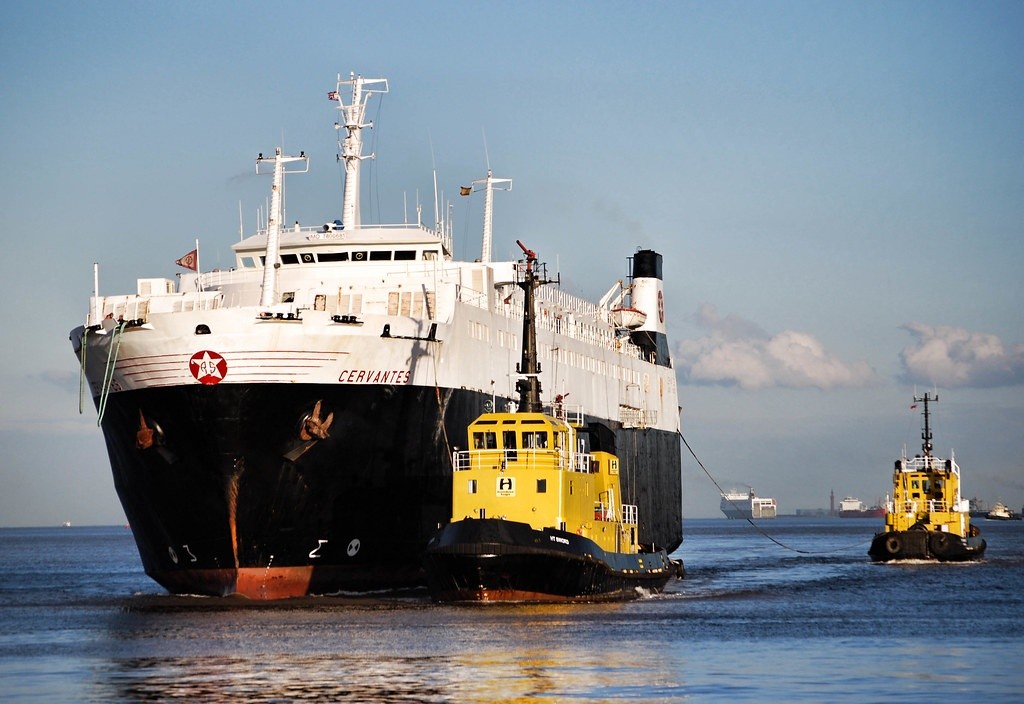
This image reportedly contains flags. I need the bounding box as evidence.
[328,91,339,101]
[459,187,472,196]
[176,249,199,271]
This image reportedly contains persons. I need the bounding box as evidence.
[106,313,125,330]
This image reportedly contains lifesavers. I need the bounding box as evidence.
[932,533,951,556]
[675,559,686,579]
[886,536,901,553]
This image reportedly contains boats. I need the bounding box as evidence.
[985,502,1021,520]
[68,70,685,599]
[419,240,685,605]
[720,486,778,518]
[867,380,987,563]
[838,492,893,518]
